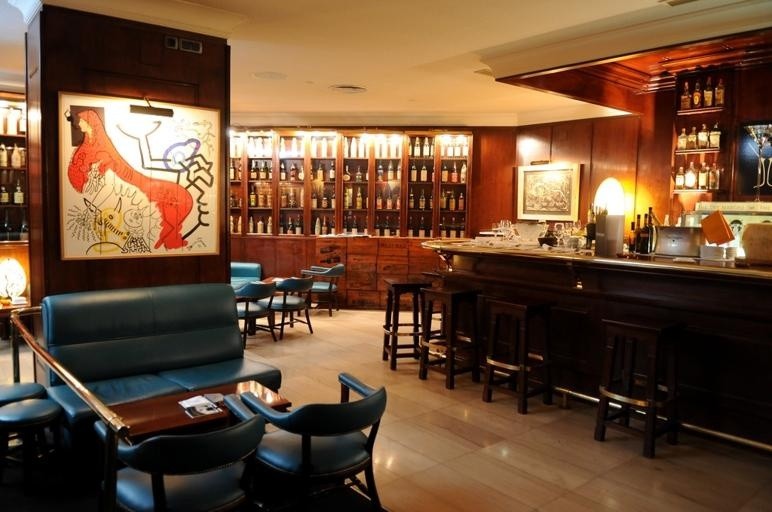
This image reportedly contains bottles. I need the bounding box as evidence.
[463,138,468,158]
[7,106,17,134]
[440,143,444,158]
[684,162,697,189]
[703,76,712,107]
[258,192,265,207]
[366,194,369,211]
[408,194,414,209]
[420,161,427,182]
[287,216,294,234]
[449,188,456,211]
[230,216,233,233]
[356,187,363,209]
[251,160,257,180]
[230,192,235,207]
[230,139,235,155]
[343,216,348,235]
[260,161,266,180]
[289,162,296,181]
[414,137,420,158]
[351,138,356,157]
[455,143,461,158]
[281,191,286,207]
[386,193,393,210]
[395,216,401,236]
[714,78,725,106]
[247,137,256,156]
[364,216,368,235]
[331,185,335,209]
[20,208,29,241]
[351,215,358,235]
[687,127,696,149]
[635,214,642,258]
[281,163,285,181]
[267,192,272,207]
[6,147,12,167]
[395,195,400,209]
[322,216,327,235]
[11,143,21,169]
[365,169,368,182]
[430,195,433,210]
[449,217,457,238]
[344,193,348,209]
[264,138,272,156]
[431,141,434,158]
[248,216,254,233]
[458,192,464,211]
[710,121,720,148]
[238,160,241,180]
[374,216,380,236]
[640,214,651,262]
[432,167,435,182]
[296,214,301,234]
[358,140,364,158]
[300,188,304,208]
[311,138,317,157]
[256,138,264,156]
[441,161,448,183]
[376,192,384,209]
[343,138,348,158]
[381,141,387,157]
[461,162,467,183]
[384,216,390,236]
[408,137,412,158]
[270,161,272,179]
[408,216,413,237]
[19,148,26,166]
[629,222,637,258]
[330,160,336,181]
[292,138,297,157]
[321,198,327,208]
[709,162,720,190]
[451,161,458,182]
[387,160,394,182]
[418,215,425,238]
[678,128,687,148]
[440,189,446,210]
[347,211,351,231]
[585,210,596,257]
[356,166,362,181]
[238,216,241,233]
[397,160,401,181]
[675,166,685,189]
[440,216,446,237]
[1,143,7,166]
[18,109,26,135]
[14,178,24,204]
[298,165,304,180]
[322,138,327,157]
[699,162,708,190]
[460,218,465,238]
[239,197,242,207]
[680,81,691,110]
[249,192,256,207]
[343,165,351,181]
[237,139,241,156]
[693,80,701,108]
[311,192,317,208]
[374,140,380,158]
[288,188,296,207]
[279,214,283,234]
[390,143,396,157]
[377,161,383,182]
[346,186,353,208]
[423,137,430,158]
[3,207,13,241]
[317,163,323,181]
[310,165,313,181]
[0,187,9,204]
[280,138,285,156]
[648,207,658,255]
[418,188,426,209]
[332,138,335,157]
[399,141,402,158]
[698,123,710,149]
[230,158,234,180]
[257,216,264,233]
[366,141,368,157]
[330,216,334,235]
[300,140,305,157]
[267,216,272,232]
[430,227,433,237]
[314,217,320,235]
[411,161,417,182]
[447,143,453,158]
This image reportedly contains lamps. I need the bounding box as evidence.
[746,125,772,202]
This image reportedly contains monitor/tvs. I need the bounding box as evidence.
[655,226,704,259]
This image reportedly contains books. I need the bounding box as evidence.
[184,401,223,419]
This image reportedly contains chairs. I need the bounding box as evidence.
[95,394,266,510]
[301,264,344,316]
[233,281,276,348]
[257,275,314,340]
[243,372,387,511]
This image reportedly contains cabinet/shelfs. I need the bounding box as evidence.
[671,71,733,198]
[231,128,472,307]
[0,92,32,243]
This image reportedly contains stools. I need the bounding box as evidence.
[594,311,679,457]
[419,283,481,389]
[383,277,431,370]
[482,294,554,415]
[0,381,45,400]
[1,400,67,468]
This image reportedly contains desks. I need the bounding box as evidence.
[106,381,292,444]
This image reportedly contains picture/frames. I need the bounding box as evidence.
[517,165,582,223]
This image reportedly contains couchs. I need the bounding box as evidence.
[40,283,285,418]
[231,263,261,289]
